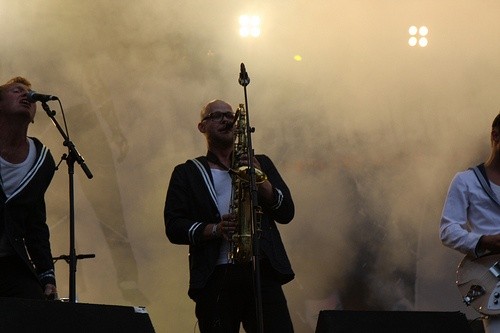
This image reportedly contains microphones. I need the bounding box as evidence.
[239,63,250,86]
[26,92,58,103]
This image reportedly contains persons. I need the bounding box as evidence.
[0,76,57,299]
[164,99,296,333]
[440,113,500,333]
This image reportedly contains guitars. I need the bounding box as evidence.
[457,248,500,314]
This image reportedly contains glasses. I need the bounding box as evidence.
[201,111,236,122]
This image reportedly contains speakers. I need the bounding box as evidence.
[0,295,155,333]
[315,310,474,333]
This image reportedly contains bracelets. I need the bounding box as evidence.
[212,223,217,236]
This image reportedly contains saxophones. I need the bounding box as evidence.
[223,103,267,276]
